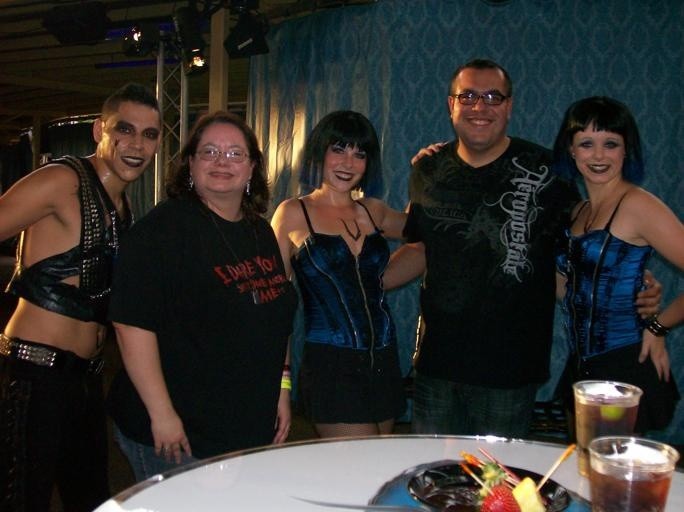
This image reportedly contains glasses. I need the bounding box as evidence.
[452,91,506,105]
[199,144,247,163]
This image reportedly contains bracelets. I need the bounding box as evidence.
[279,364,291,391]
[646,317,671,338]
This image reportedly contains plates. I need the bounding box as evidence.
[408,464,572,512]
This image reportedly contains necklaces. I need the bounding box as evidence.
[208,200,262,307]
[584,205,602,234]
[337,216,363,243]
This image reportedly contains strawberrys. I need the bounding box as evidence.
[481,486,520,511]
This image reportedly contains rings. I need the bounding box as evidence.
[163,447,172,454]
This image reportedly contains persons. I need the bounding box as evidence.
[0,83,160,510]
[112,104,296,484]
[271,110,448,439]
[383,57,666,441]
[554,95,684,453]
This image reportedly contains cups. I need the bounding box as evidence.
[587,434,680,512]
[572,380,644,479]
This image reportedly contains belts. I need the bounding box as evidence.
[1,334,105,376]
